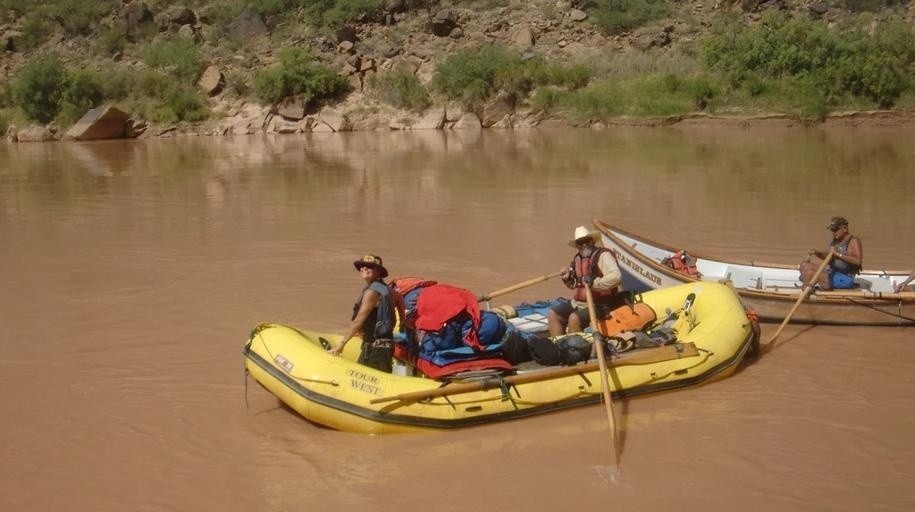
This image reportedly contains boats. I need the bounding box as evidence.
[242,280,755,436]
[593,218,910,325]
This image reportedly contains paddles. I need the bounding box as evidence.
[581,276,620,469]
[368,341,700,404]
[763,252,834,348]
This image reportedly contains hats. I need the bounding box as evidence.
[826,217,848,230]
[354,254,388,277]
[566,226,601,247]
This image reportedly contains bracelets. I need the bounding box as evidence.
[839,253,843,258]
[340,339,345,345]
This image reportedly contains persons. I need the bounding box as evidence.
[547,226,623,339]
[800,216,864,288]
[329,251,406,373]
